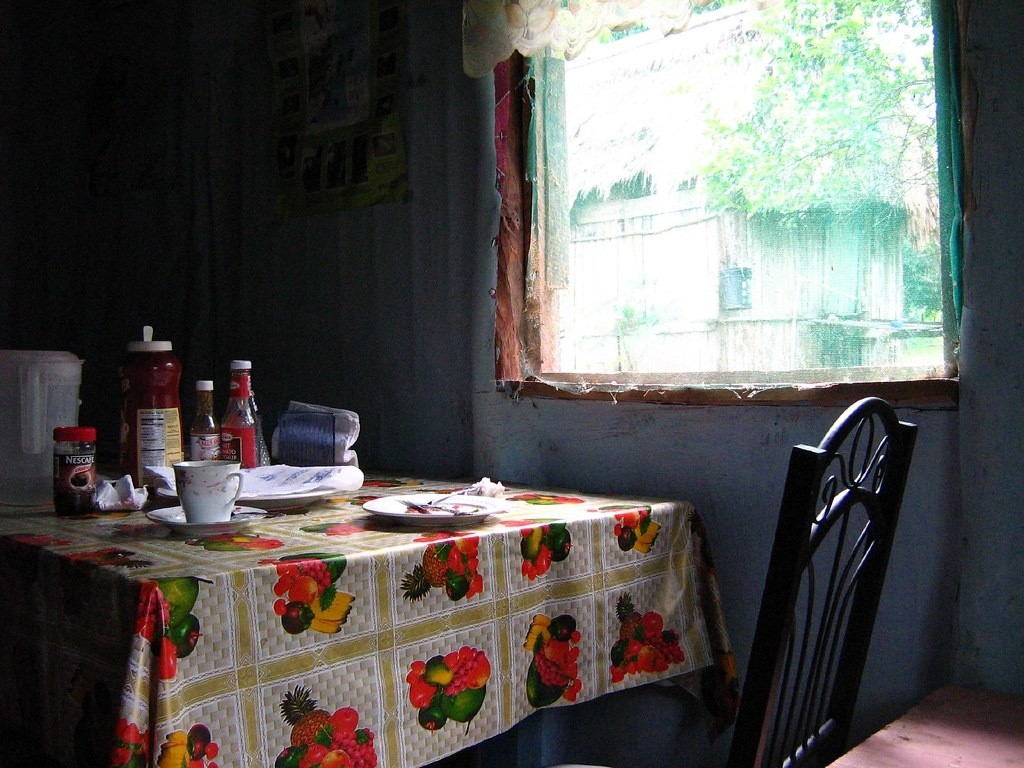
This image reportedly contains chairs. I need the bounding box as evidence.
[721,395,919,768]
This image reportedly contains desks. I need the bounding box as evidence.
[0,465,739,768]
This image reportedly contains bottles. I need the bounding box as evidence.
[220,360,270,468]
[189,381,220,461]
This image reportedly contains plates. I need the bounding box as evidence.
[156,465,364,512]
[145,505,268,536]
[362,494,510,526]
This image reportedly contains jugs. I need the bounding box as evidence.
[0,349,86,506]
